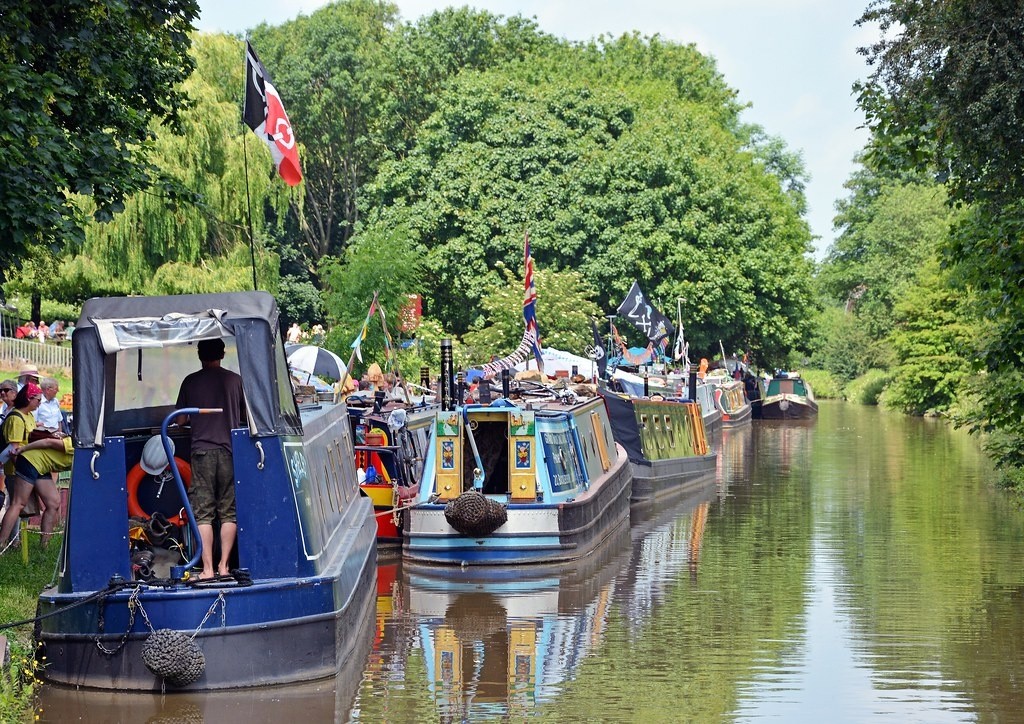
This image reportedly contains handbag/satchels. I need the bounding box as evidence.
[28,429,60,443]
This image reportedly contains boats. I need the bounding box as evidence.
[348,296,821,568]
[34,578,381,724]
[400,514,635,723]
[32,290,380,693]
[631,476,719,544]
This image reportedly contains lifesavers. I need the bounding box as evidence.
[126,457,192,528]
[648,378,665,385]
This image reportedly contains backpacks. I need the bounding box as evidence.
[0,411,26,454]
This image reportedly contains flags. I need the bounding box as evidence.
[592,321,607,379]
[523,233,543,364]
[241,39,302,187]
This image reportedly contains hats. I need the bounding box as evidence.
[13,365,44,379]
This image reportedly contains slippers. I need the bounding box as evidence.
[219,573,232,579]
[187,576,214,584]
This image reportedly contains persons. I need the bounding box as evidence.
[731,367,766,401]
[0,365,75,551]
[175,338,247,582]
[359,372,407,402]
[24,320,76,346]
[286,322,324,343]
[469,377,479,392]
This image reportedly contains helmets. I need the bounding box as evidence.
[140,434,175,475]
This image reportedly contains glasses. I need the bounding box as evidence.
[33,397,41,402]
[31,377,38,381]
[0,388,12,393]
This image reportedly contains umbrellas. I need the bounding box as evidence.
[285,345,347,385]
